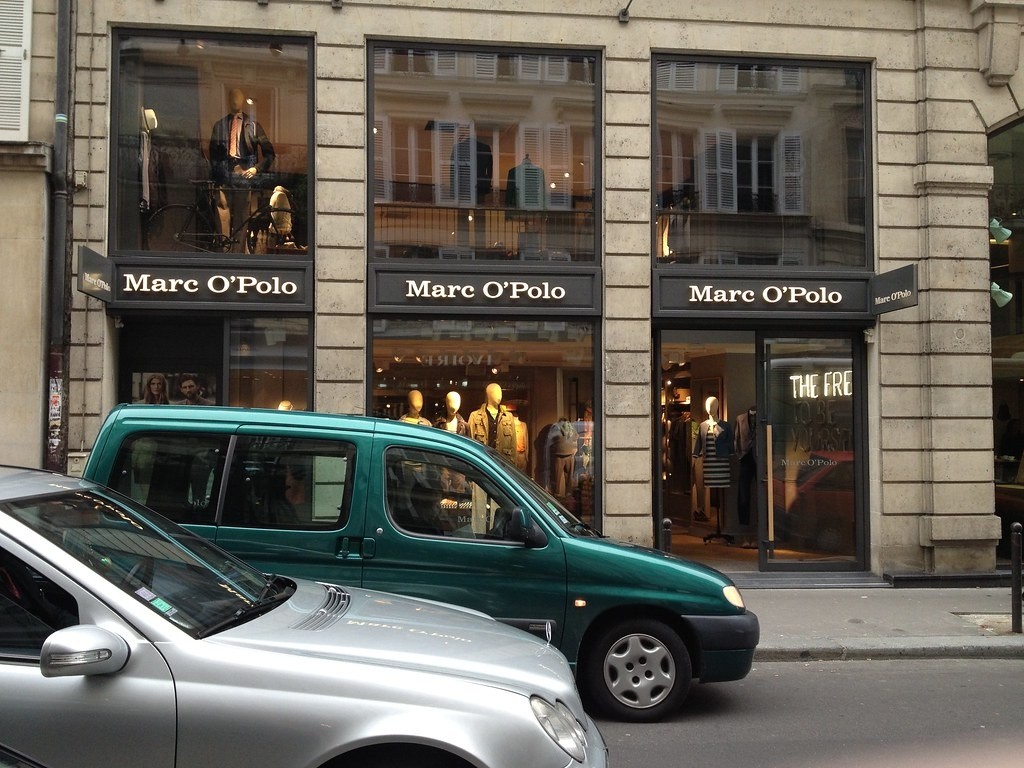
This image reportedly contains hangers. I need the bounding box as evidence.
[679,412,692,421]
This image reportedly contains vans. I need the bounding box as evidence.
[64,402,760,724]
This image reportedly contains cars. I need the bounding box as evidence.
[0,464,611,768]
[751,450,855,552]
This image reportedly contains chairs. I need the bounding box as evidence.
[238,454,301,525]
[410,479,443,530]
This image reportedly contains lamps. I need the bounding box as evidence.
[989,216,1012,243]
[661,352,672,370]
[668,351,686,366]
[989,282,1013,307]
[491,364,503,374]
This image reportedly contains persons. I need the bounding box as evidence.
[210,88,292,254]
[450,126,493,247]
[692,397,758,525]
[277,400,306,505]
[131,373,216,509]
[504,154,544,221]
[548,417,579,499]
[580,445,593,473]
[395,383,529,496]
